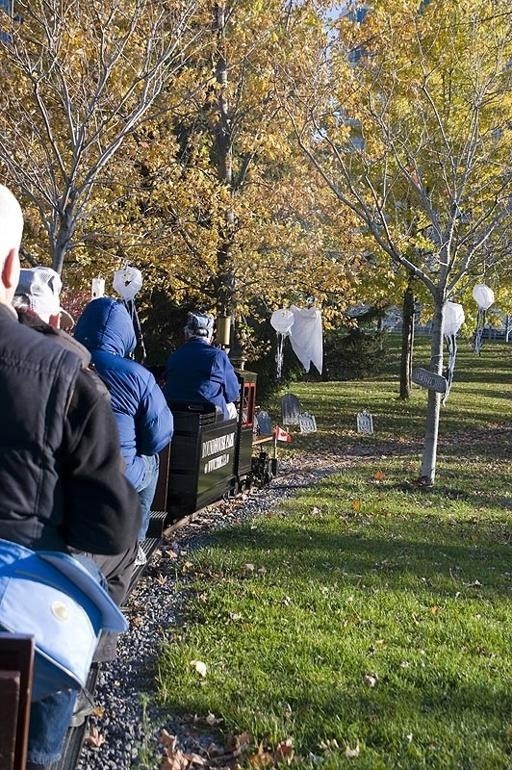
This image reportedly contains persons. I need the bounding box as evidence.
[21,268,138,725]
[1,183,138,769]
[72,298,173,566]
[163,312,240,423]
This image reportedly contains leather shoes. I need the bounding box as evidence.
[70,690,95,727]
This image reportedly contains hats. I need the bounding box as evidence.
[17,265,74,329]
[187,314,214,336]
